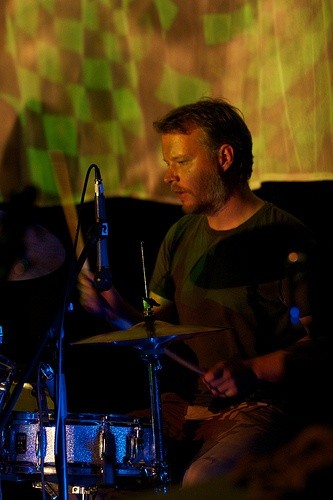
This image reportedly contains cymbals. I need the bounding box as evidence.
[0,220,66,281]
[0,381,56,411]
[70,319,233,345]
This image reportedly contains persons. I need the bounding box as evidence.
[79,101,312,487]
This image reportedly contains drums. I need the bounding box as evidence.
[1,414,166,475]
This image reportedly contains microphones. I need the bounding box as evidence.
[94,168,112,290]
